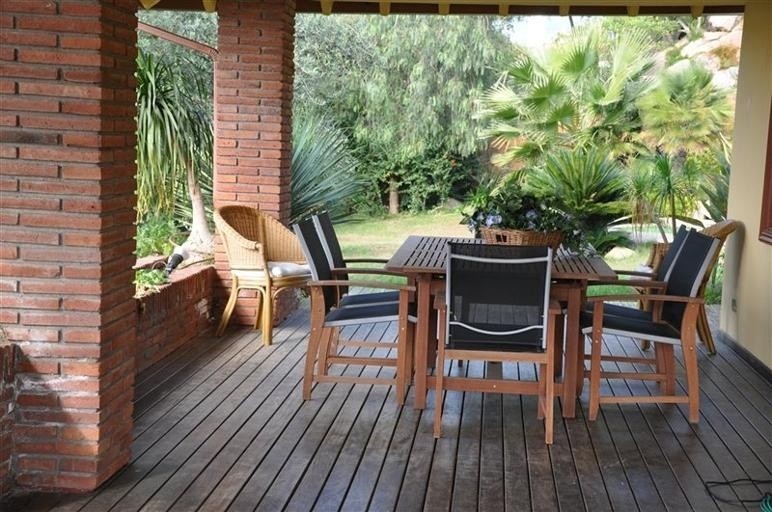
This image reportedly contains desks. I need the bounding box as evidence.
[385,235,619,417]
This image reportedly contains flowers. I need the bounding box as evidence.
[461,171,579,252]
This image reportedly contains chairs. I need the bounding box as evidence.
[293,220,417,405]
[639,219,737,354]
[576,228,719,423]
[587,225,694,390]
[214,205,311,345]
[313,211,417,356]
[433,245,561,444]
